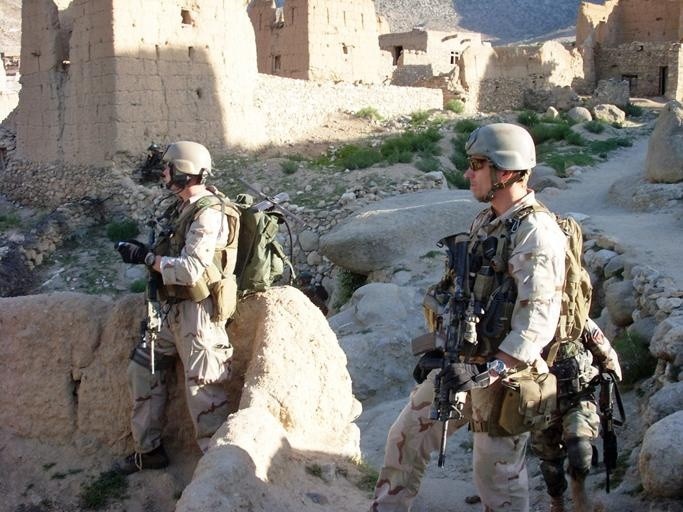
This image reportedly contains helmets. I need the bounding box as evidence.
[463,122,537,172]
[161,139,212,176]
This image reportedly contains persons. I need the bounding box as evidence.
[111,141,238,476]
[528,315,621,511]
[368,123,566,510]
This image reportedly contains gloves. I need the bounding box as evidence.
[435,360,491,394]
[114,236,149,264]
[412,351,445,386]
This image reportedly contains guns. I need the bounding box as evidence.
[411,233,494,467]
[599,371,618,495]
[129,219,177,375]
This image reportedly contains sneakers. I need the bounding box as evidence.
[116,443,169,475]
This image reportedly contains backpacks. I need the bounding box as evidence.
[169,191,296,299]
[499,204,595,348]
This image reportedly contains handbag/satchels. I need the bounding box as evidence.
[208,274,238,321]
[499,361,562,437]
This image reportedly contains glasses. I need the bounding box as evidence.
[464,155,489,171]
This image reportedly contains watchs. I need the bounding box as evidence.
[490,359,508,374]
